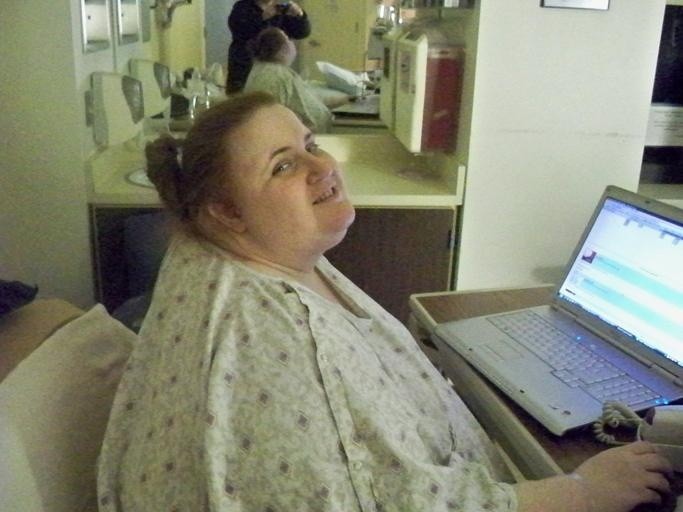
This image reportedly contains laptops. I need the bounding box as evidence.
[432,186,683,437]
[331,96,379,119]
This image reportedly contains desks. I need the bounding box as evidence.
[404,285,683,512]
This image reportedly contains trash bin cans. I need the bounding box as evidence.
[112,290,154,336]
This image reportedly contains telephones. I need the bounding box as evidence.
[636,405,683,471]
[358,94,380,107]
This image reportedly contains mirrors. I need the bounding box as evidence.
[72,0,401,134]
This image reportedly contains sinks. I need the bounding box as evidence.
[126,167,155,189]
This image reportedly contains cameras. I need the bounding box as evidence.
[275,2,290,12]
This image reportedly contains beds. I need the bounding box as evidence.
[0,296,137,511]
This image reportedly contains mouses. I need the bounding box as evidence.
[630,487,677,511]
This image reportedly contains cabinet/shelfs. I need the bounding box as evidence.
[93,209,459,314]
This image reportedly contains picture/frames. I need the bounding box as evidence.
[540,0,610,11]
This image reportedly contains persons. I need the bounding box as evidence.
[224,0,334,133]
[98,93,675,511]
